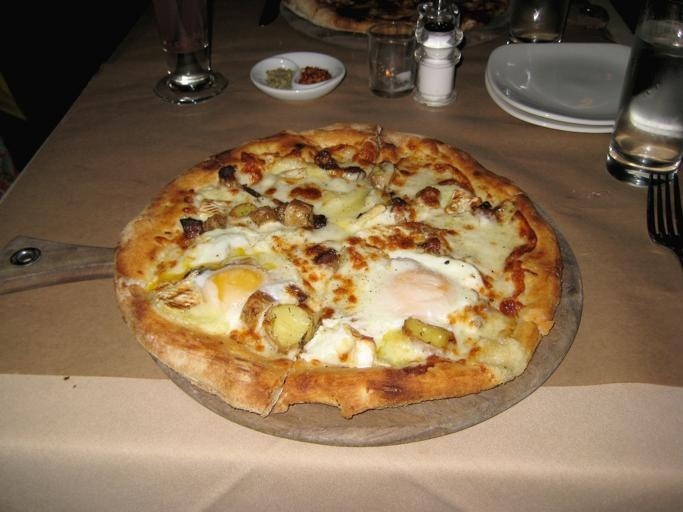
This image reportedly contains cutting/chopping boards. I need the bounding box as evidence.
[1,125,585,448]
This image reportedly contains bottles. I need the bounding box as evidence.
[414,22,465,110]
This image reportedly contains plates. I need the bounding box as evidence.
[483,42,641,136]
[246,50,346,101]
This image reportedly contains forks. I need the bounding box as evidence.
[645,168,682,281]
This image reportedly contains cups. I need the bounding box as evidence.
[601,2,682,186]
[152,0,229,104]
[366,21,418,98]
[502,0,570,44]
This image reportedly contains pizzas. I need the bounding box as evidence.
[281,0,510,38]
[114,121,563,418]
